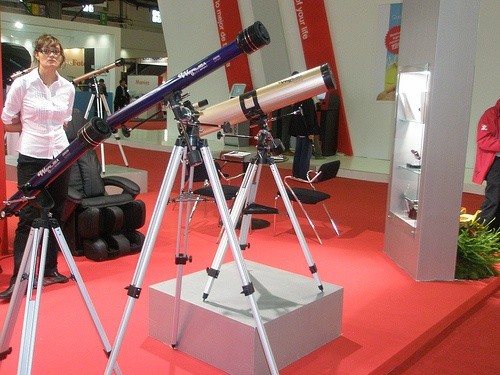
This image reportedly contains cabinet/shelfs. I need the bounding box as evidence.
[384,0,481,282]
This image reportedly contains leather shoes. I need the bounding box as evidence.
[44,271,69,283]
[0,282,27,298]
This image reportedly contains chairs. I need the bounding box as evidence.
[273,160,342,245]
[63,108,147,262]
[187,160,241,233]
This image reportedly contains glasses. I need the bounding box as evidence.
[38,47,63,54]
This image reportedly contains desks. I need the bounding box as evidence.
[221,151,289,231]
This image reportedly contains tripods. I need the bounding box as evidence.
[202,114,324,301]
[0,186,123,375]
[102,89,279,375]
[84,76,129,175]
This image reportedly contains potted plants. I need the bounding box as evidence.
[454,206,500,280]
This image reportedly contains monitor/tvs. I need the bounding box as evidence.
[230,84,246,97]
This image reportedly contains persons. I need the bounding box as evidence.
[113,79,130,126]
[281,71,325,182]
[470,98,500,232]
[0,31,76,298]
[93,79,107,119]
